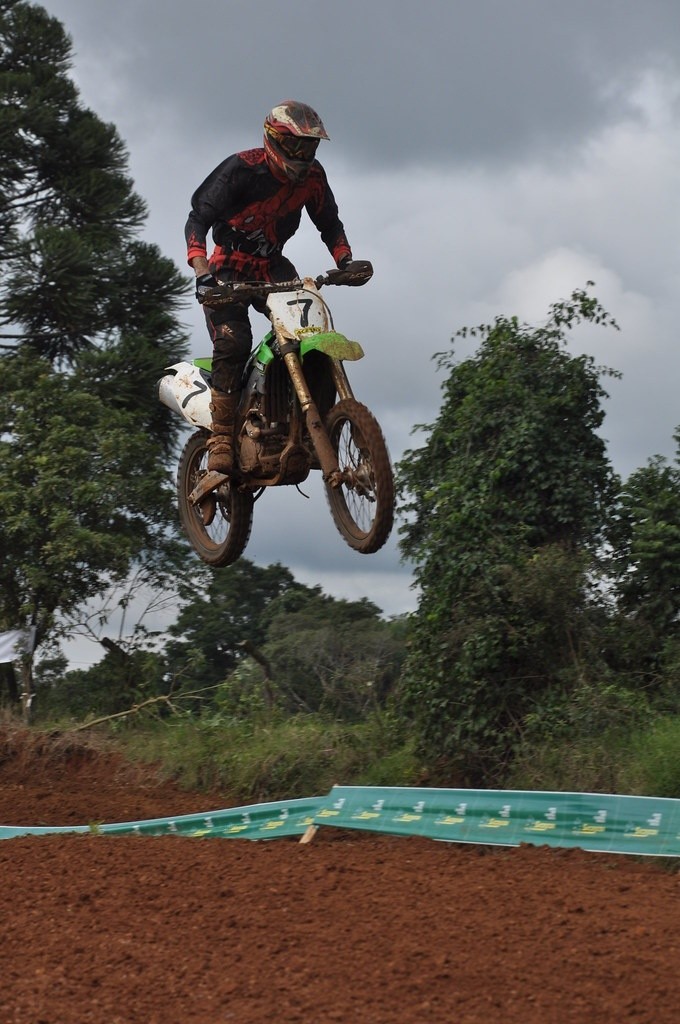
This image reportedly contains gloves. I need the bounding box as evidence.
[340,260,353,272]
[196,274,222,305]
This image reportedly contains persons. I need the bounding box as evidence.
[184,101,373,470]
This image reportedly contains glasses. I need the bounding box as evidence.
[279,135,319,159]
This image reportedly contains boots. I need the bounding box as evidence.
[206,388,238,473]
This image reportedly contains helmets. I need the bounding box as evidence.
[263,101,330,185]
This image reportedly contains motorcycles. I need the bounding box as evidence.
[158,264,399,569]
